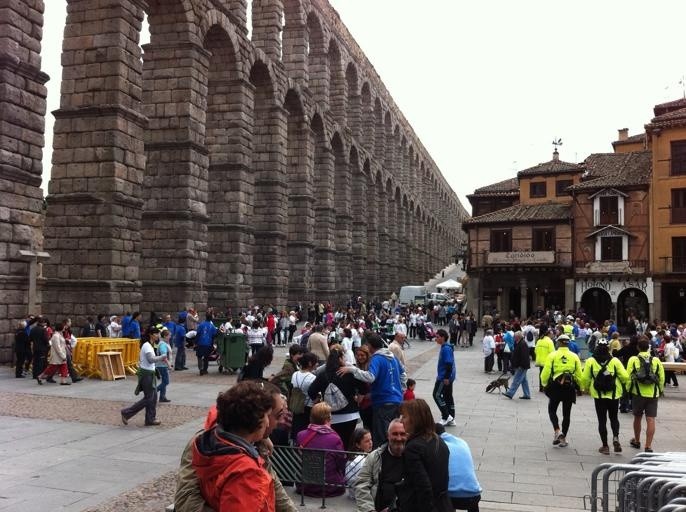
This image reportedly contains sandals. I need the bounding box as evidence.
[37,377,42,385]
[60,382,71,385]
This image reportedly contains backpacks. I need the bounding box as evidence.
[626,338,664,452]
[588,363,625,401]
[546,371,582,405]
[524,327,535,343]
[320,371,348,416]
[288,372,310,413]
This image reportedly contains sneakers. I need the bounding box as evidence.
[613,439,622,453]
[159,398,170,402]
[645,447,653,452]
[438,414,453,426]
[121,410,128,425]
[630,439,641,449]
[599,446,609,454]
[145,419,160,425]
[446,420,456,426]
[553,431,563,445]
[559,438,568,447]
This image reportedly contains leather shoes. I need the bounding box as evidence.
[519,396,531,399]
[182,367,188,369]
[72,377,84,383]
[502,392,512,399]
[174,368,183,371]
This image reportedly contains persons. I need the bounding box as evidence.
[225,317,233,330]
[180,307,188,321]
[272,315,283,346]
[449,291,465,307]
[493,311,534,330]
[502,331,530,400]
[627,311,664,359]
[665,337,678,390]
[584,339,634,457]
[15,321,28,379]
[69,331,78,349]
[31,314,49,379]
[664,321,685,345]
[268,312,275,344]
[470,315,475,348]
[270,340,304,391]
[122,328,167,428]
[299,322,365,348]
[502,324,515,375]
[269,395,290,448]
[290,353,317,409]
[339,329,356,369]
[469,308,473,315]
[121,313,144,341]
[81,317,97,337]
[370,296,394,330]
[388,330,417,399]
[523,320,538,360]
[35,323,72,385]
[463,316,470,348]
[395,316,409,338]
[346,426,372,499]
[290,311,297,341]
[20,315,36,369]
[432,330,458,425]
[482,328,498,374]
[336,329,403,450]
[429,295,451,324]
[294,400,348,497]
[537,334,584,448]
[307,326,330,365]
[458,312,466,343]
[415,306,427,345]
[109,316,120,337]
[97,314,110,337]
[43,318,53,340]
[260,320,268,349]
[396,299,429,318]
[47,318,84,383]
[281,312,290,344]
[311,342,347,374]
[354,418,407,512]
[235,311,255,326]
[175,318,189,371]
[481,311,492,333]
[185,308,198,347]
[495,327,505,370]
[249,322,263,354]
[401,399,450,512]
[166,313,175,362]
[208,305,214,318]
[196,312,218,375]
[226,305,232,317]
[534,301,622,357]
[452,299,460,314]
[248,304,272,324]
[297,294,369,322]
[409,309,418,336]
[434,422,482,512]
[310,347,368,456]
[174,379,295,512]
[354,345,367,373]
[154,315,165,330]
[190,380,276,511]
[448,311,459,350]
[156,330,173,404]
[29,314,42,375]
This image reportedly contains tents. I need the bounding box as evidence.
[436,278,462,293]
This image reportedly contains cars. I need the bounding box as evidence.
[428,293,454,303]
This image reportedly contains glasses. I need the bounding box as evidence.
[269,412,283,420]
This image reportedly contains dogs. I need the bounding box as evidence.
[485,375,511,394]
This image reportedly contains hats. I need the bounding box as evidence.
[555,335,570,341]
[597,338,608,346]
